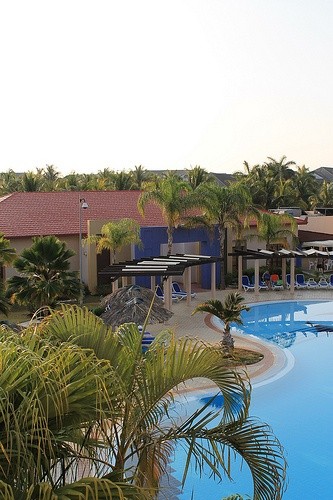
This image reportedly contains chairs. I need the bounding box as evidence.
[286,274,308,289]
[241,271,283,293]
[154,282,196,302]
[307,274,333,288]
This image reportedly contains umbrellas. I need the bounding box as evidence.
[100,285,166,309]
[98,297,174,330]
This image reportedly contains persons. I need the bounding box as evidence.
[263,271,270,291]
[271,273,280,290]
[322,259,325,274]
[324,257,328,270]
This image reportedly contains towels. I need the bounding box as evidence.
[277,279,282,284]
[271,274,278,281]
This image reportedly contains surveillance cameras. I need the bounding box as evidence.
[82,203,88,209]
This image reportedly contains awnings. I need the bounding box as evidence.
[301,240,333,249]
